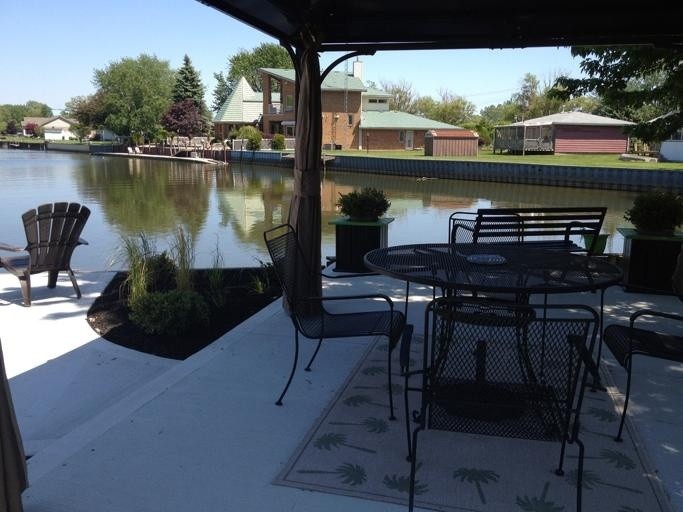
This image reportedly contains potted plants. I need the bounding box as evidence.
[623,187,683,236]
[335,187,392,222]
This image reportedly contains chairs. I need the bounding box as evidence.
[406,296,600,512]
[590,308,683,443]
[262,223,405,421]
[0,202,90,307]
[426,208,609,382]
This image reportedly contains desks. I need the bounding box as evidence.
[362,241,625,417]
